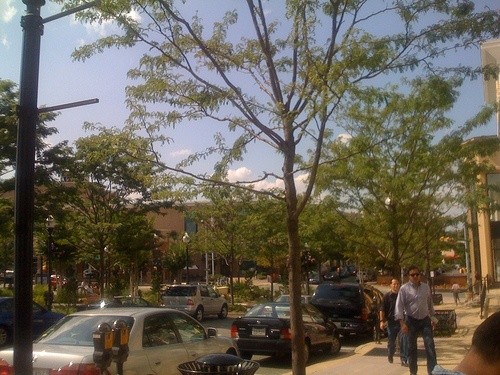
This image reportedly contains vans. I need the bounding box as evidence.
[309,281,388,343]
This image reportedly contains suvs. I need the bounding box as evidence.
[0,270,15,284]
[356,271,368,282]
[50,274,77,291]
[163,282,228,322]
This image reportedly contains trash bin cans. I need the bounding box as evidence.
[433,310,452,336]
[178,353,260,375]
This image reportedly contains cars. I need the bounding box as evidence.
[275,294,311,303]
[0,306,239,375]
[309,265,357,284]
[230,302,342,364]
[86,295,155,308]
[0,296,69,347]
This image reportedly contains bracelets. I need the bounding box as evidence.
[380,319,384,322]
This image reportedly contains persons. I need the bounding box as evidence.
[432,312,500,375]
[394,265,438,375]
[452,281,462,305]
[379,277,409,367]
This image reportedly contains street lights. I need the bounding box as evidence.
[183,232,191,284]
[104,244,114,296]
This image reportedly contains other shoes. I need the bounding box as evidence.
[388,356,393,363]
[401,360,409,367]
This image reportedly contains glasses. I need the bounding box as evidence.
[409,273,419,277]
